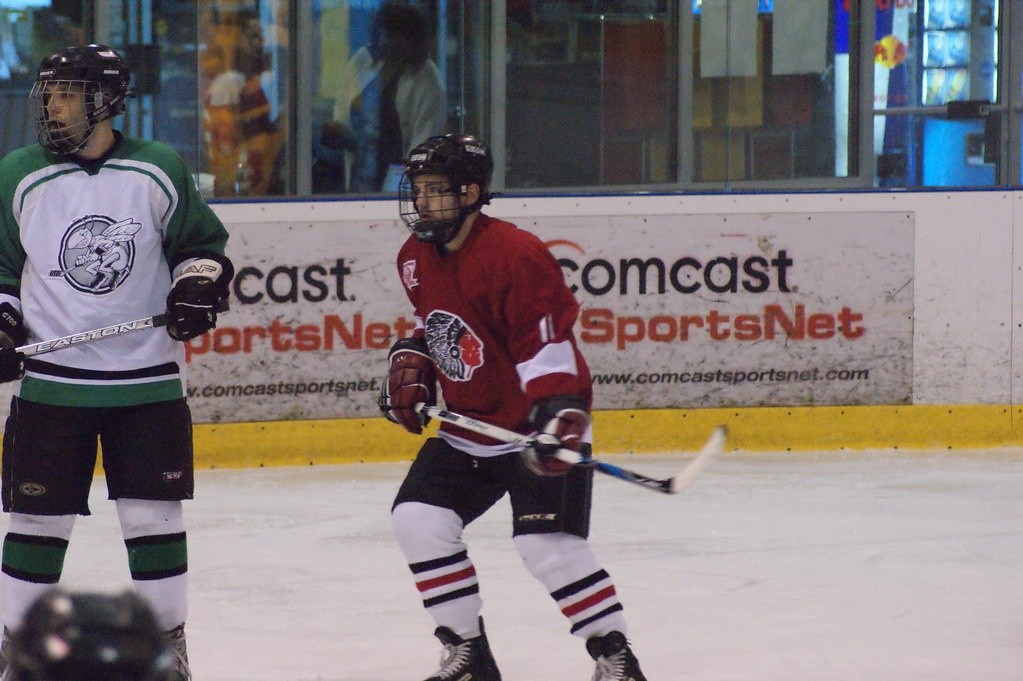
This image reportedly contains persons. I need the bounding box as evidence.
[378,133,647,681]
[0,587,176,681]
[319,0,445,195]
[205,12,287,197]
[0,45,234,681]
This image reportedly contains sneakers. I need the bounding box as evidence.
[585,631,647,681]
[0,625,16,681]
[159,622,192,681]
[421,615,503,681]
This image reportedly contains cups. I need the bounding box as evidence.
[199,175,214,197]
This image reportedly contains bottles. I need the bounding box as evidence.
[232,150,254,197]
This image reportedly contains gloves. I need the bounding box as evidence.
[165,250,235,341]
[524,393,593,477]
[376,337,433,434]
[0,284,30,383]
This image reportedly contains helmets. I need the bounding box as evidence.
[10,588,172,681]
[405,133,494,244]
[37,44,133,158]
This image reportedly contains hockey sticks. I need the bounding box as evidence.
[0,298,231,357]
[414,399,730,495]
